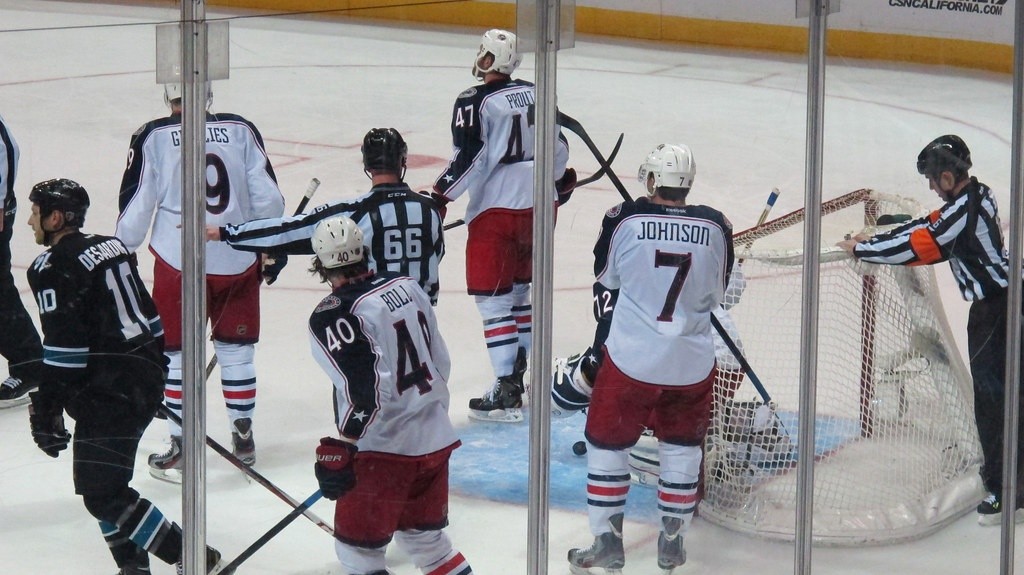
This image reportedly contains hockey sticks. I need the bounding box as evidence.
[217,489,321,575]
[738,186,781,267]
[557,112,775,408]
[205,175,321,379]
[442,131,625,232]
[160,403,410,569]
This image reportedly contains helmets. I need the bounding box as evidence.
[917,135,972,177]
[361,127,408,170]
[29,179,89,227]
[165,80,212,101]
[310,216,364,269]
[642,143,696,198]
[476,29,525,76]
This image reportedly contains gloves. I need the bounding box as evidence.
[261,253,288,287]
[158,354,171,397]
[554,168,577,205]
[28,391,72,459]
[416,184,451,224]
[314,436,359,501]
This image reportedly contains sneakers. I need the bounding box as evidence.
[568,512,626,575]
[156,521,237,575]
[468,362,525,423]
[657,516,687,575]
[234,418,256,485]
[150,435,183,484]
[977,486,1024,526]
[0,375,41,409]
[114,551,151,575]
[511,346,530,406]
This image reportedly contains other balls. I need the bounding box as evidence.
[572,441,587,456]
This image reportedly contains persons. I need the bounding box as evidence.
[836,134,1024,526]
[28,178,236,575]
[0,116,45,407]
[308,216,475,575]
[567,143,735,575]
[113,83,285,484]
[206,127,446,306]
[433,28,577,423]
